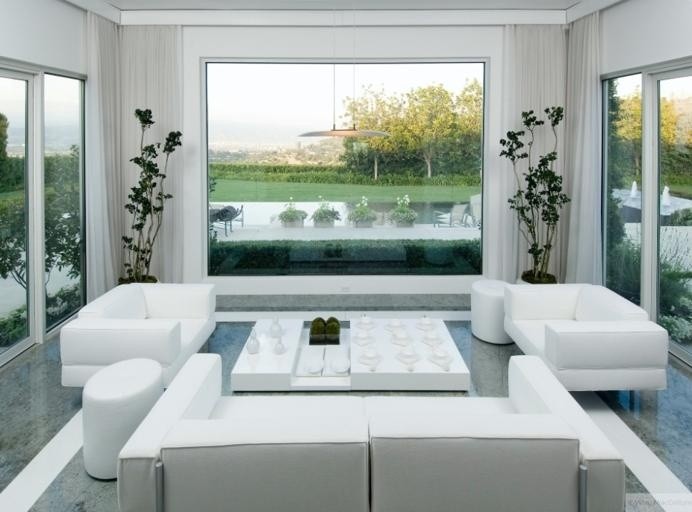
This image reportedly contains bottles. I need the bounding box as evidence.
[269,313,288,355]
[246,327,260,354]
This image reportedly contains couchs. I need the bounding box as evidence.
[117,353,626,512]
[503,283,668,392]
[59,283,217,390]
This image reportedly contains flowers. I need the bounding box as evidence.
[389,195,416,224]
[348,196,377,222]
[311,195,341,223]
[279,196,307,221]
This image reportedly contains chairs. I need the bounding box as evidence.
[433,194,482,228]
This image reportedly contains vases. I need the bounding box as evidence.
[315,221,334,228]
[354,221,373,228]
[280,220,304,228]
[396,221,415,228]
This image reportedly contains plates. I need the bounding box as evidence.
[353,312,456,372]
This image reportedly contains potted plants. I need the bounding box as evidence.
[119,110,183,283]
[499,106,571,284]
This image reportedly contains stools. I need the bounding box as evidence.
[82,359,163,481]
[471,280,514,345]
